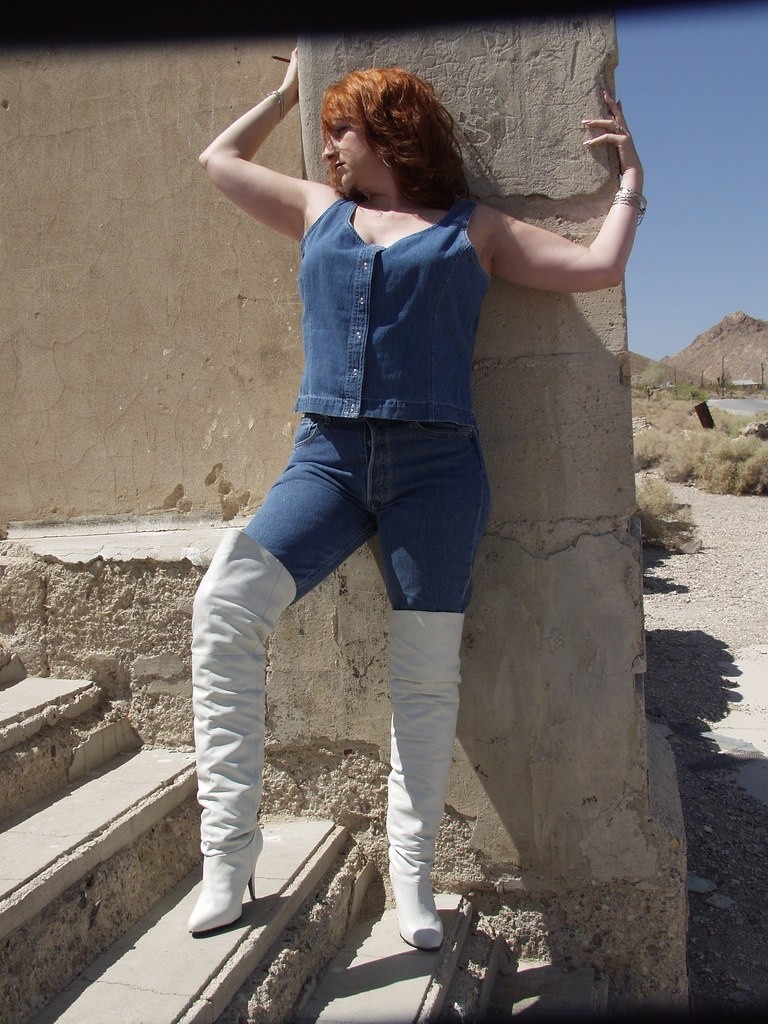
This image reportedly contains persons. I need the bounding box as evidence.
[186,38,647,950]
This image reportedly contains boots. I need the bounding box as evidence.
[188,528,296,933]
[386,610,465,952]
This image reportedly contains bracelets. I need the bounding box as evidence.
[270,91,285,122]
[610,185,647,226]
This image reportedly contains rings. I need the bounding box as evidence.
[615,126,624,134]
